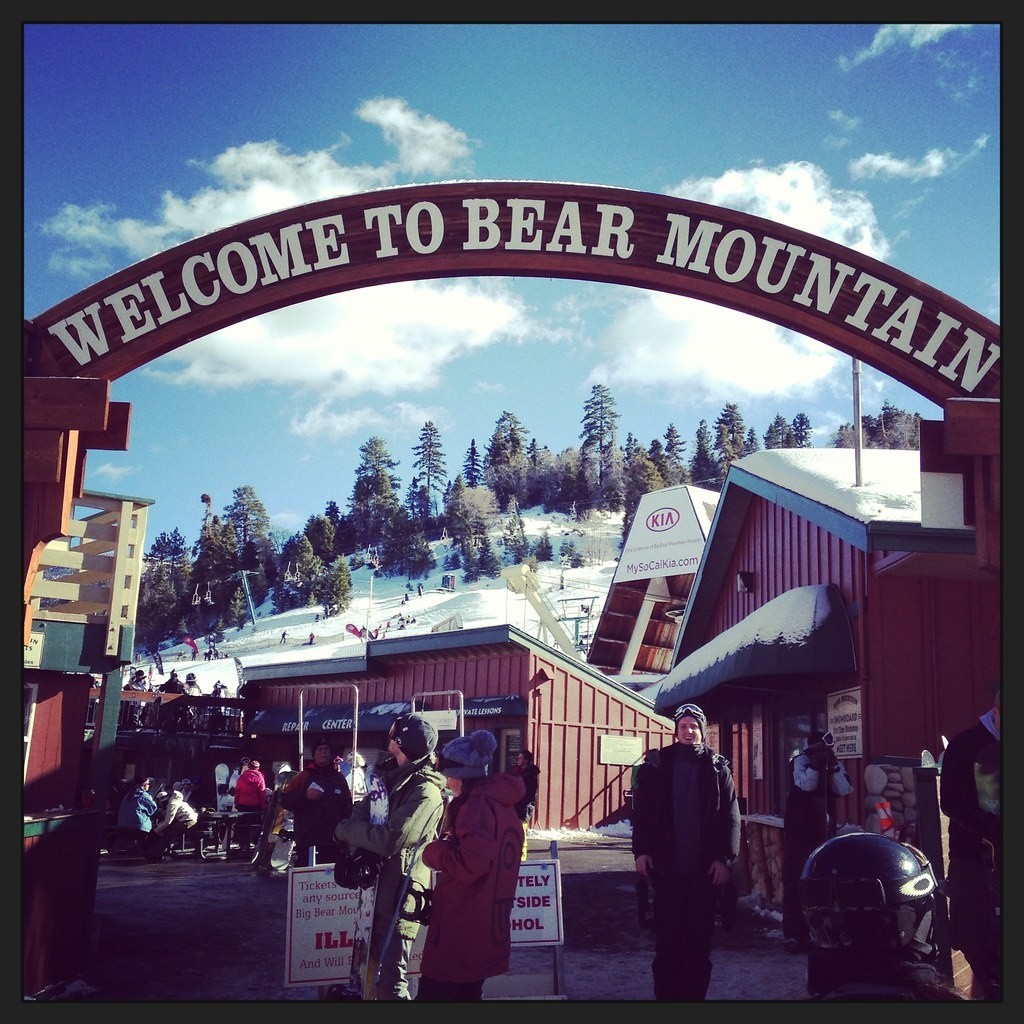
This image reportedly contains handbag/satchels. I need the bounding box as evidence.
[334,844,381,890]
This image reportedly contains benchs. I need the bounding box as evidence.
[164,830,213,859]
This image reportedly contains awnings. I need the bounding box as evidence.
[652,581,861,724]
[244,697,431,741]
[453,694,528,719]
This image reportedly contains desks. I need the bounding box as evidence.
[198,811,258,851]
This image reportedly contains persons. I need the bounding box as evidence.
[779,730,856,958]
[797,831,978,1000]
[579,640,586,654]
[630,702,744,1000]
[938,688,1003,1000]
[277,581,427,646]
[104,736,391,871]
[513,751,542,863]
[131,629,231,664]
[410,735,527,1001]
[325,714,449,1001]
[63,668,239,732]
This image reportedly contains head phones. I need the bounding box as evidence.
[396,712,414,747]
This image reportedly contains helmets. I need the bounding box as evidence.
[798,832,938,949]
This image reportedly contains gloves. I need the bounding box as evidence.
[808,753,825,771]
[826,749,838,766]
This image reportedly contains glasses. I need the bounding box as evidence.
[433,751,465,772]
[822,731,836,746]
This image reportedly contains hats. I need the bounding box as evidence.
[334,756,343,762]
[311,738,330,758]
[279,763,292,775]
[673,704,707,741]
[807,732,826,743]
[441,730,497,779]
[396,712,436,761]
